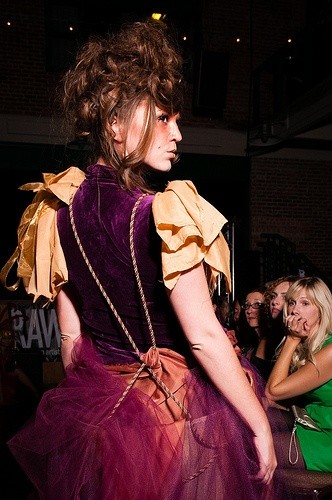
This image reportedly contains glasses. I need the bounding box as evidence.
[242,302,263,310]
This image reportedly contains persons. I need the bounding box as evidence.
[0,320,41,434]
[0,22,278,500]
[213,272,332,500]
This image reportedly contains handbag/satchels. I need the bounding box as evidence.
[289,404,321,465]
